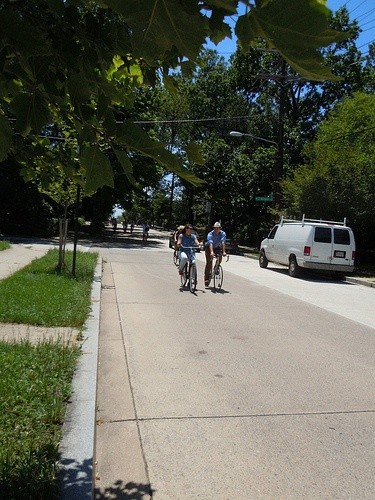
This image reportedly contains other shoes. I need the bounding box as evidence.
[179,270,184,275]
[205,280,209,287]
[215,269,219,274]
[191,279,197,285]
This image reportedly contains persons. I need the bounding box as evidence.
[123,220,127,232]
[177,224,203,283]
[204,222,227,287]
[111,217,117,230]
[143,221,148,240]
[174,225,185,257]
[131,221,134,231]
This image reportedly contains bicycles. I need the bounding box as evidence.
[142,233,148,245]
[130,225,134,233]
[179,244,201,293]
[124,225,127,233]
[113,225,117,233]
[204,253,230,290]
[173,240,185,266]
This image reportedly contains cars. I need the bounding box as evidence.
[169,230,201,253]
[110,217,116,223]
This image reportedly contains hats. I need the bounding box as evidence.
[213,222,222,228]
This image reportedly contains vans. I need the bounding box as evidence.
[259,213,356,279]
